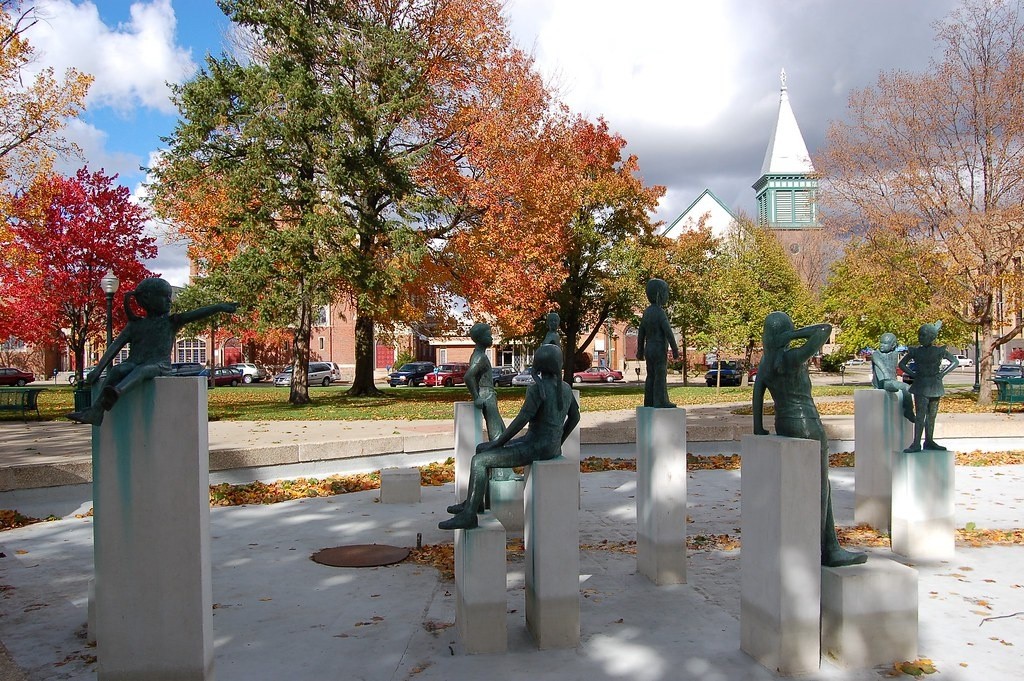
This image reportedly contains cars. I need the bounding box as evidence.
[846,359,863,365]
[68,367,107,384]
[902,362,916,383]
[705,360,743,387]
[573,366,623,384]
[739,362,757,382]
[994,364,1024,380]
[0,368,36,387]
[491,366,518,387]
[511,366,542,386]
[214,363,266,384]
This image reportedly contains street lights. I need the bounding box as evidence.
[971,296,981,391]
[101,269,119,372]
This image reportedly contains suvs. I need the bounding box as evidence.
[945,355,973,367]
[424,363,469,387]
[197,367,242,388]
[171,362,203,377]
[273,363,333,388]
[321,362,341,383]
[387,361,435,388]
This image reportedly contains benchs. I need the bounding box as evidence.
[0,389,49,423]
[986,378,1024,415]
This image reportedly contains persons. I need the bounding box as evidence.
[66,277,240,427]
[752,311,868,567]
[542,313,560,348]
[636,279,679,408]
[870,333,916,425]
[463,323,517,480]
[898,321,958,452]
[437,342,581,531]
